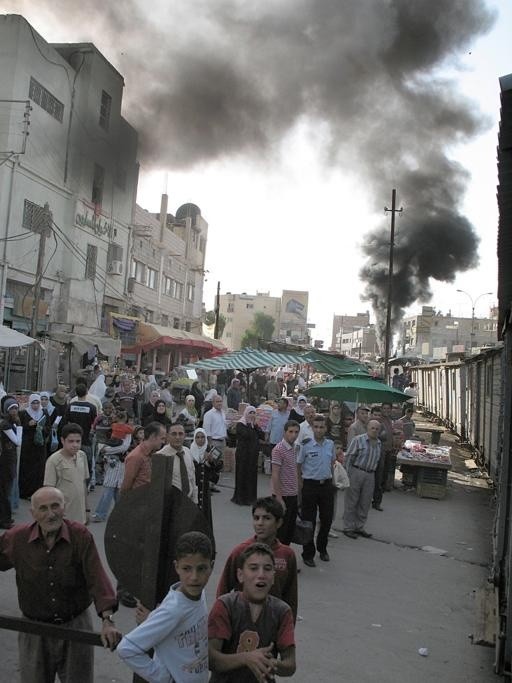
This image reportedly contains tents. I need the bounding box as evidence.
[139,322,227,356]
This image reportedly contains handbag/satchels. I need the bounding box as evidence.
[49,428,58,452]
[290,512,313,546]
[33,427,44,447]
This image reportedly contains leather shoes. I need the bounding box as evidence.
[117,590,137,607]
[304,559,315,566]
[354,528,372,537]
[210,488,221,492]
[344,531,358,538]
[372,504,384,511]
[320,552,329,561]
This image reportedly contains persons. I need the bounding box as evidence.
[399,408,416,473]
[91,405,131,523]
[156,422,199,508]
[173,395,198,449]
[105,412,135,468]
[105,375,124,412]
[217,365,286,410]
[70,377,102,491]
[141,374,172,433]
[287,365,314,397]
[0,486,124,683]
[114,422,166,608]
[296,415,338,568]
[92,402,114,468]
[393,368,404,392]
[207,544,296,683]
[342,406,370,522]
[133,375,143,422]
[1,399,23,529]
[401,382,419,418]
[117,531,216,683]
[266,398,291,479]
[231,405,265,506]
[371,407,387,512]
[288,395,307,422]
[18,394,49,502]
[188,428,219,525]
[58,384,96,512]
[49,385,67,457]
[380,402,393,492]
[191,381,204,423]
[127,425,145,460]
[89,374,107,404]
[40,390,49,451]
[203,395,226,493]
[341,420,382,539]
[269,420,301,573]
[214,495,299,629]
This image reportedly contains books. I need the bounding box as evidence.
[37,415,47,426]
[203,446,221,468]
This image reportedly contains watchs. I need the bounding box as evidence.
[102,614,116,623]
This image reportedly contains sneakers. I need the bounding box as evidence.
[328,532,338,538]
[1,523,15,529]
[90,485,94,491]
[10,518,14,523]
[89,514,104,522]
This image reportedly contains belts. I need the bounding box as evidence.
[305,478,329,484]
[176,451,190,495]
[22,600,93,625]
[213,438,224,441]
[352,464,373,473]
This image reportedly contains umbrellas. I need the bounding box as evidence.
[176,346,322,371]
[0,321,47,353]
[304,377,416,423]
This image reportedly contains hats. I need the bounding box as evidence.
[357,405,370,414]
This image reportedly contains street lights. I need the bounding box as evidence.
[456,290,494,353]
